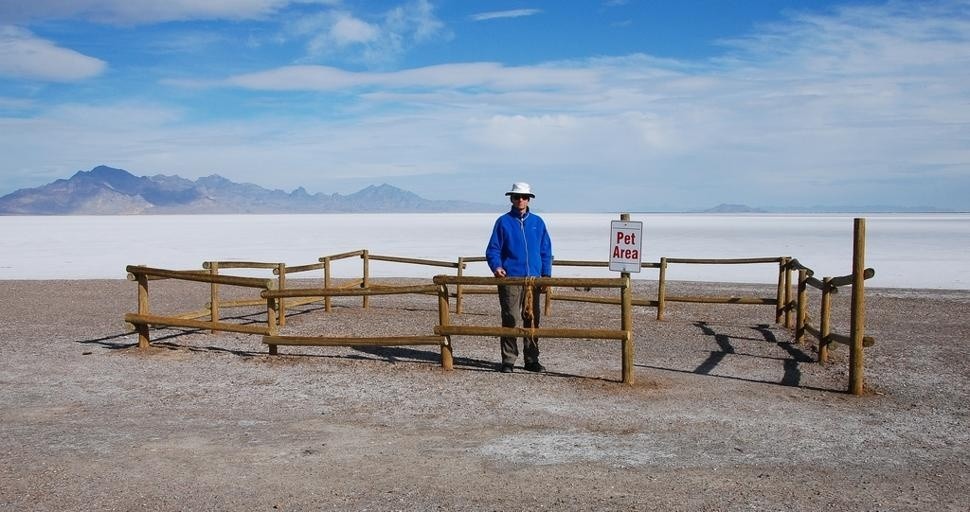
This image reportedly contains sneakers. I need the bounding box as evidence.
[499,361,514,373]
[524,362,547,373]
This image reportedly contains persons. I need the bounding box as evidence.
[484,181,553,375]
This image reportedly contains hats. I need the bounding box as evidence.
[505,183,535,199]
